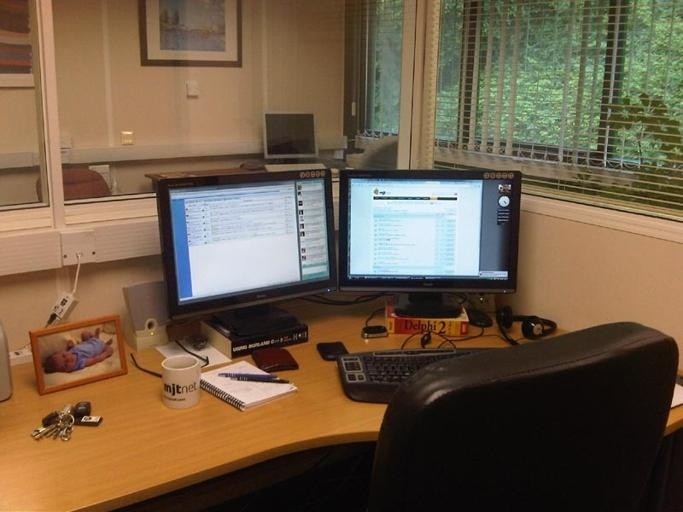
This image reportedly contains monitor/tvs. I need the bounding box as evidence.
[339,168,521,318]
[261,111,319,159]
[155,168,337,336]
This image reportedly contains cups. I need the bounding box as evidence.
[161,356,201,408]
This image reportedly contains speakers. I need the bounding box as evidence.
[122,279,171,351]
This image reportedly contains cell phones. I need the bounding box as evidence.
[317,342,348,360]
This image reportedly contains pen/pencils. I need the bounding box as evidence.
[218,373,289,383]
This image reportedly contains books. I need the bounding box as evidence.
[198,359,298,410]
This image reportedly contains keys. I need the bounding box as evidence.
[31,400,104,442]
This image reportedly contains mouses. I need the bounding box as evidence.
[331,167,339,174]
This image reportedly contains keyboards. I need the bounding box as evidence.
[264,163,327,172]
[337,348,490,403]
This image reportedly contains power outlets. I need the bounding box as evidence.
[60,229,93,267]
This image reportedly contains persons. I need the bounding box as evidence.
[42,327,113,374]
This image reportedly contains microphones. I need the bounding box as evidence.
[498,324,517,346]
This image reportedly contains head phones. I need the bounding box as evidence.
[496,304,557,340]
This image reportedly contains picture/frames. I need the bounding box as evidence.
[29,314,127,397]
[138,0,242,68]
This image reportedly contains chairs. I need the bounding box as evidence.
[36,169,112,203]
[368,321,678,512]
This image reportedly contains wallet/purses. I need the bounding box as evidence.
[252,348,299,372]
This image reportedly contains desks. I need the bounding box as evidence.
[144,169,266,192]
[1,293,683,512]
